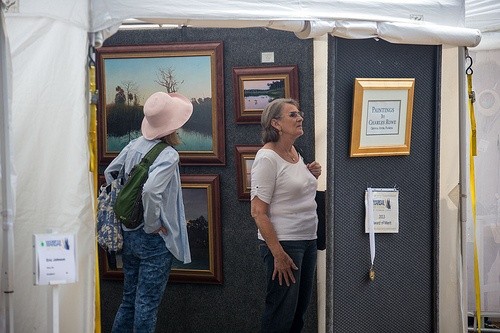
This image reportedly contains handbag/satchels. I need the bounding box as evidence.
[96,148,124,253]
[114,142,172,229]
[294,143,327,250]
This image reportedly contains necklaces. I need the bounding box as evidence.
[276,141,295,162]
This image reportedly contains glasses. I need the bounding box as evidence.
[275,111,306,119]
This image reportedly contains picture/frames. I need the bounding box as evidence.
[232,64,299,125]
[235,145,264,201]
[102,172,222,282]
[95,41,226,166]
[350,78,416,157]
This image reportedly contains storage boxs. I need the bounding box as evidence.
[467,310,500,333]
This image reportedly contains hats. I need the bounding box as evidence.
[141,91,194,141]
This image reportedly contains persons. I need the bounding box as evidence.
[250,98,322,333]
[104,91,193,333]
